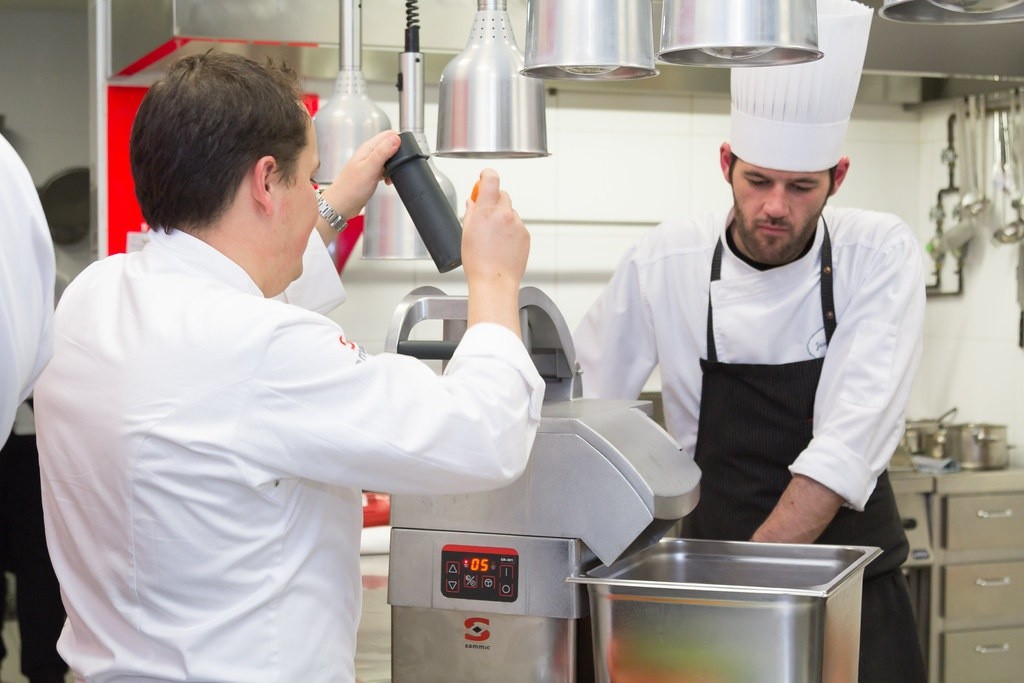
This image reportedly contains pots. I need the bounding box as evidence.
[904,420,1009,471]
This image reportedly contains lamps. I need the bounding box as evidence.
[517,0,660,82]
[364,0,457,260]
[877,1,1024,27]
[311,0,390,184]
[654,0,824,69]
[435,0,549,159]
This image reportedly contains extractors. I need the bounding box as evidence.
[108,0,1024,122]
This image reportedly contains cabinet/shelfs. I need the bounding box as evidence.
[942,493,1024,683]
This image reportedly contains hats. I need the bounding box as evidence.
[730,0,874,172]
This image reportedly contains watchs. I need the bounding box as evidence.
[316,192,347,232]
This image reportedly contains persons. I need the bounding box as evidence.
[34,53,546,683]
[0,132,56,452]
[573,0,930,683]
